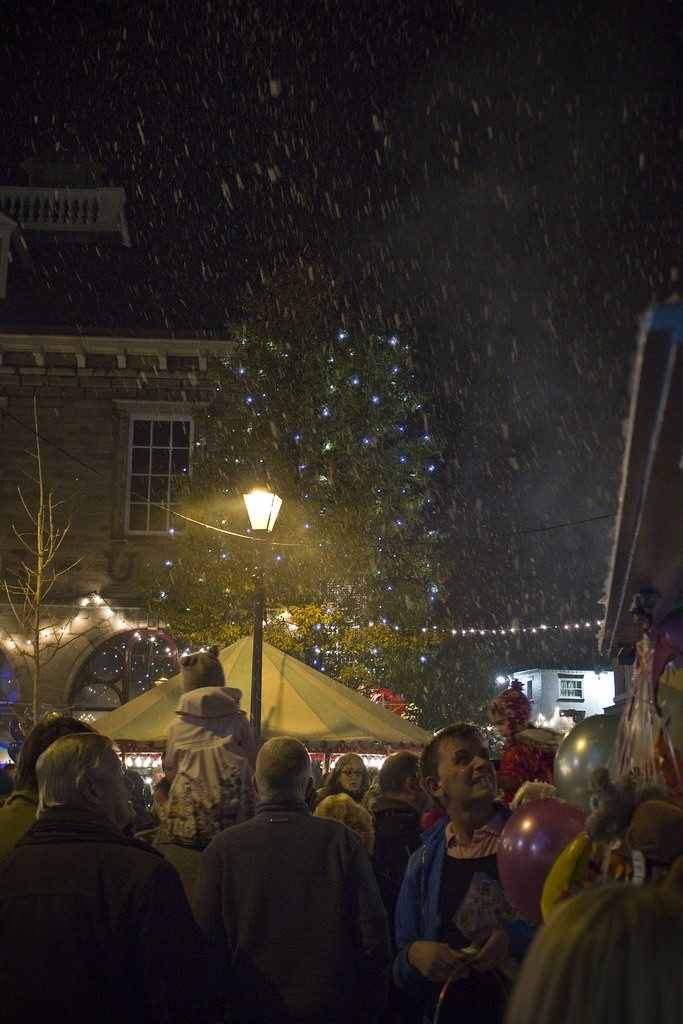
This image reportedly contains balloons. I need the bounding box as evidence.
[553,715,621,813]
[497,797,590,929]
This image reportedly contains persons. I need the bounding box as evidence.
[0,648,683,1024]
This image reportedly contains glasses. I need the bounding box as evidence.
[341,768,365,779]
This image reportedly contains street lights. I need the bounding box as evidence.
[244,460,284,739]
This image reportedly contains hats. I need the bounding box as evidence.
[177,645,223,694]
[490,679,530,726]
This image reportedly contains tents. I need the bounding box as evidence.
[91,635,435,752]
